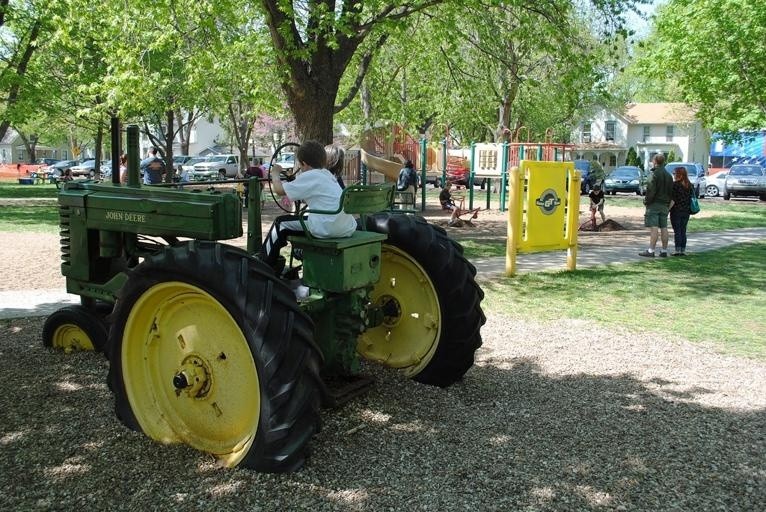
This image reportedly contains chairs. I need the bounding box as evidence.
[450,185,466,211]
[288,180,397,293]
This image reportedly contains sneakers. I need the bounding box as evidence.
[659,251,667,257]
[638,250,655,257]
[681,252,687,256]
[671,252,679,256]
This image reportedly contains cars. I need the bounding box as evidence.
[419,175,436,190]
[606,166,648,196]
[33,151,297,182]
[706,171,727,198]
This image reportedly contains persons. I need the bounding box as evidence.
[257,138,357,268]
[638,154,673,258]
[258,162,266,207]
[118,152,128,185]
[47,164,59,184]
[588,184,606,228]
[63,168,73,181]
[667,166,696,256]
[437,181,458,226]
[139,145,165,184]
[241,158,263,208]
[396,159,418,215]
[175,163,189,192]
[323,141,346,189]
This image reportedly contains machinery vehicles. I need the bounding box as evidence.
[43,124,488,473]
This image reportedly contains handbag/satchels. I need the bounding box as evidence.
[690,183,700,214]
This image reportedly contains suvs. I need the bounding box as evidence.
[567,159,605,196]
[439,144,488,189]
[724,164,766,201]
[650,160,708,200]
